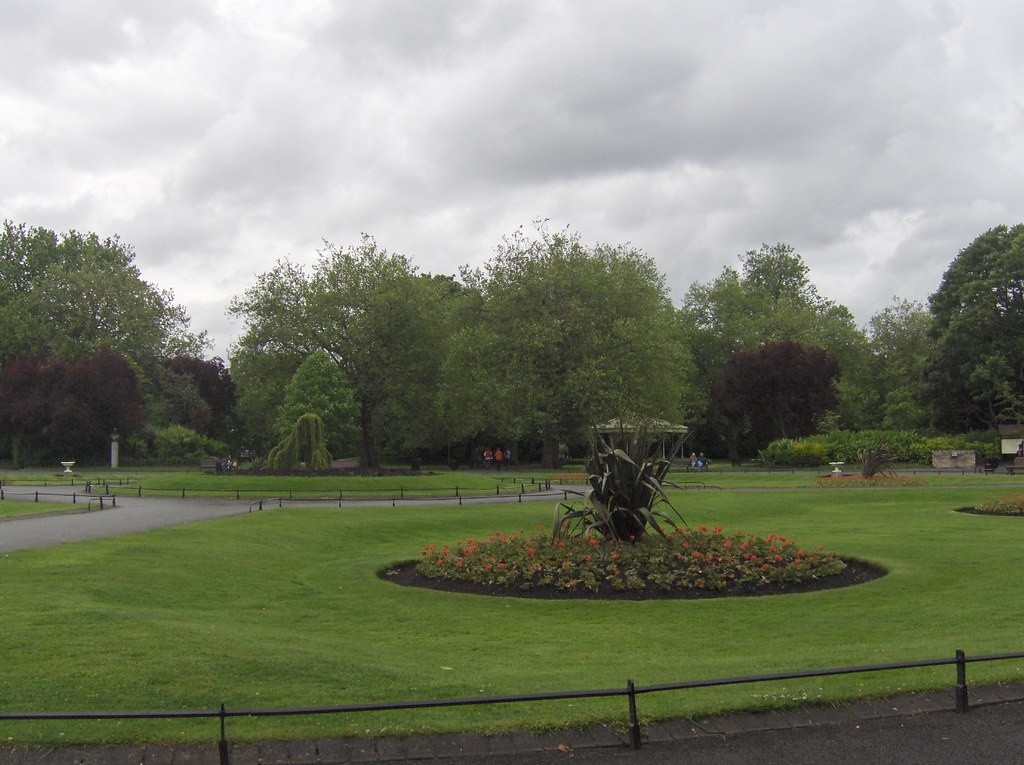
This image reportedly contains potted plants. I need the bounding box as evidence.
[829,457,844,472]
[60,453,75,472]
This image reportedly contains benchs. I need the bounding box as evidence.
[1005,456,1024,475]
[199,458,226,472]
[686,461,708,470]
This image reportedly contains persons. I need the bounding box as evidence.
[504,449,510,467]
[494,447,502,470]
[690,453,706,472]
[484,448,492,469]
[221,453,233,471]
